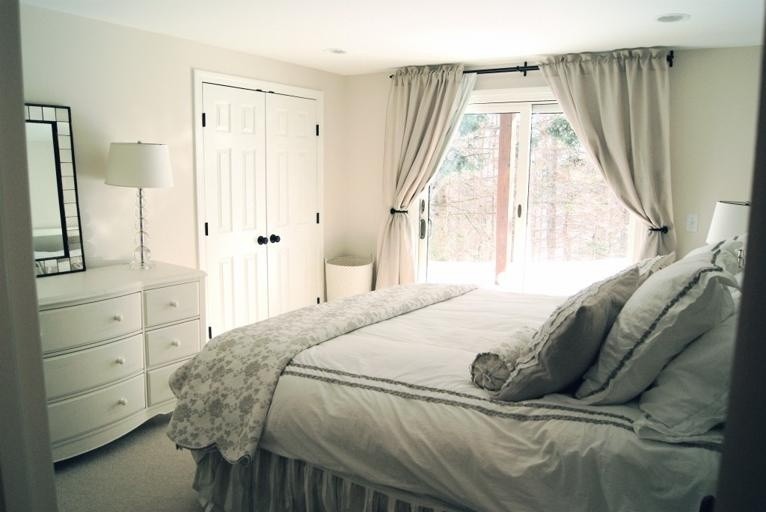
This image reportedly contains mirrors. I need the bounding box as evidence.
[23,103,88,277]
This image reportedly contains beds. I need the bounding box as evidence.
[188,241,743,512]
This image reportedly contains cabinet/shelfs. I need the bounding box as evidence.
[32,261,208,464]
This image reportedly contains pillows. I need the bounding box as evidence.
[630,282,742,439]
[572,243,739,407]
[494,264,644,402]
[468,325,538,392]
[626,248,677,286]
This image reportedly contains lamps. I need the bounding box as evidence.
[104,142,167,271]
[707,200,750,243]
